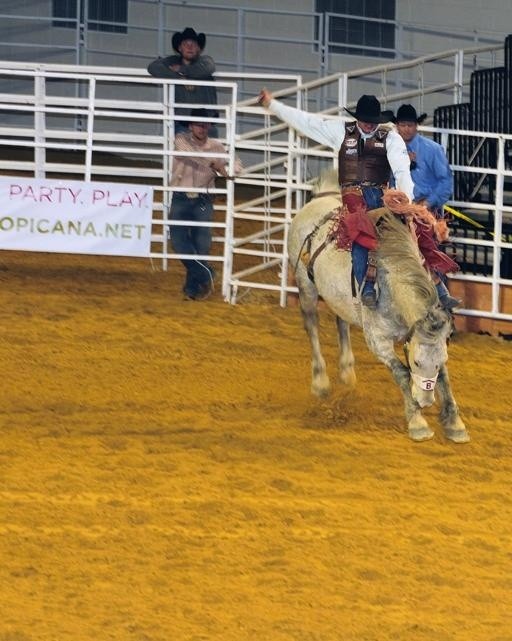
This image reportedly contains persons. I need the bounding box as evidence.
[169,108,241,303]
[147,26,221,141]
[396,104,453,282]
[258,88,462,309]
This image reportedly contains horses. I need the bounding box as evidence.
[286,166,472,445]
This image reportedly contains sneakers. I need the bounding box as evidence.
[363,290,377,313]
[180,270,218,302]
[439,293,463,308]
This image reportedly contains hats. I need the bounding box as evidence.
[171,26,206,54]
[344,94,395,124]
[178,108,220,126]
[391,102,427,124]
